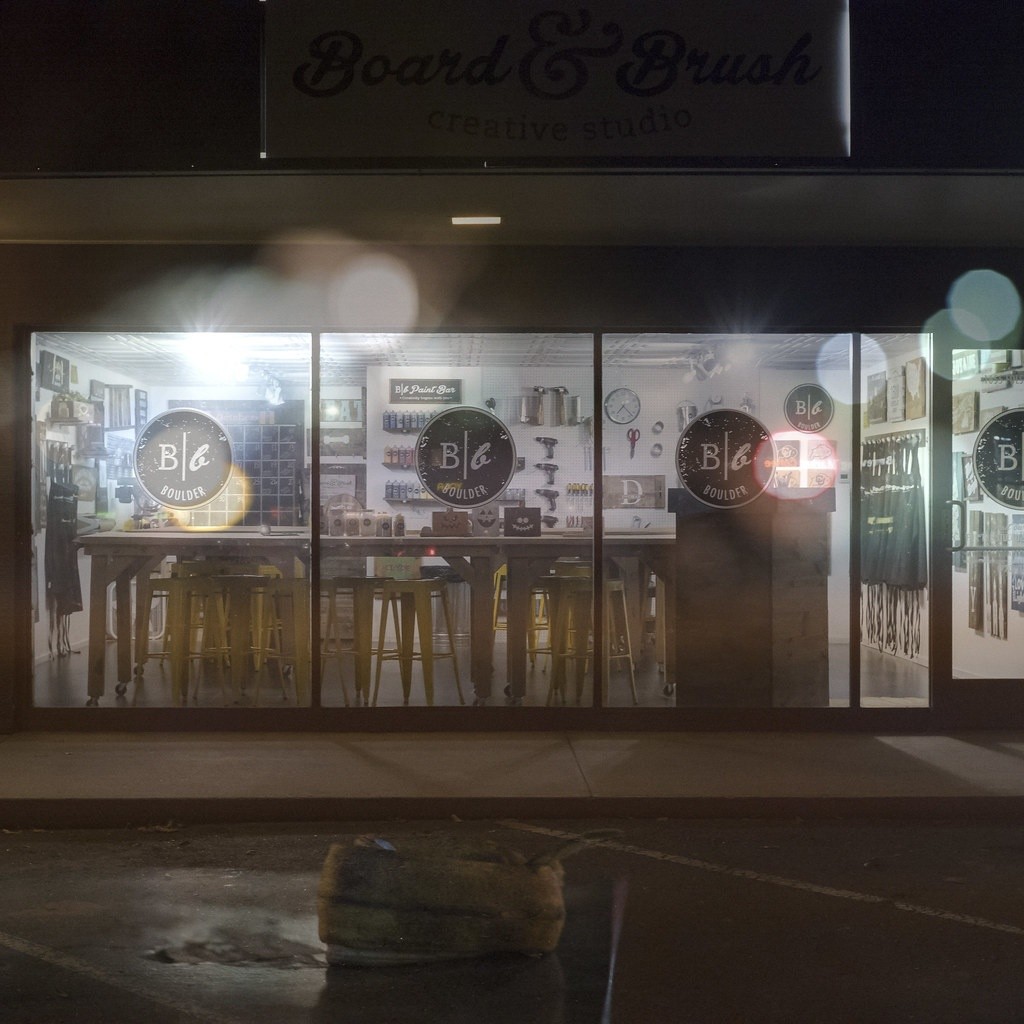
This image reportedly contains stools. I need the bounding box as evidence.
[372,576,466,706]
[548,560,621,674]
[165,577,233,706]
[545,577,638,708]
[193,573,290,701]
[253,577,351,707]
[322,576,406,707]
[493,572,552,674]
[132,576,170,680]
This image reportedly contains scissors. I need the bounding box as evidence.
[627,428,640,459]
[485,398,496,416]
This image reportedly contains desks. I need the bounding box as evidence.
[80,532,675,707]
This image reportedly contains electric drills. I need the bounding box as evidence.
[535,488,559,512]
[536,437,558,459]
[534,463,559,485]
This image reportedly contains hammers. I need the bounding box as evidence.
[533,385,547,425]
[553,386,570,425]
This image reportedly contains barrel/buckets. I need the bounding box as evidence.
[521,396,540,425]
[538,385,565,428]
[676,400,697,434]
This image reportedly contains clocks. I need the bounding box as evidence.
[604,388,641,425]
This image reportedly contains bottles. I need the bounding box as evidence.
[392,512,404,537]
[376,511,392,537]
[385,480,434,499]
[344,509,360,537]
[360,509,375,538]
[384,444,415,464]
[562,393,581,427]
[383,410,437,429]
[328,508,345,537]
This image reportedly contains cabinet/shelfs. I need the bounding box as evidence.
[190,423,299,525]
[381,427,436,504]
[45,416,116,459]
